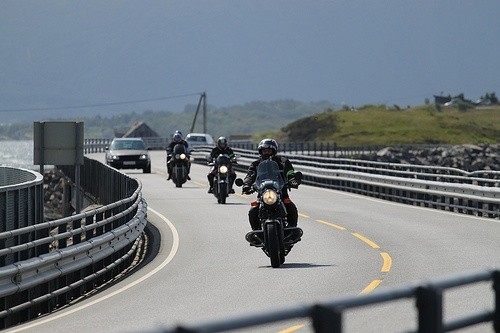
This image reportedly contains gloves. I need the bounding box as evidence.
[242,184,251,194]
[288,179,299,191]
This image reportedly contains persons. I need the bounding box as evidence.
[166,131,191,181]
[207,136,237,194]
[242,139,302,246]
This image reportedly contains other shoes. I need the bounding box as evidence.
[166,174,172,181]
[250,228,262,246]
[208,186,213,193]
[229,189,235,194]
[187,176,191,180]
[285,225,301,241]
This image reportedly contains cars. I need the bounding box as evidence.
[185,133,216,153]
[443,99,462,109]
[105,137,151,173]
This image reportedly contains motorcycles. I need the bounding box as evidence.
[234,158,304,269]
[166,143,194,187]
[206,151,241,204]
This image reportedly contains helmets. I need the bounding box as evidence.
[173,131,182,138]
[216,137,228,151]
[258,139,278,158]
